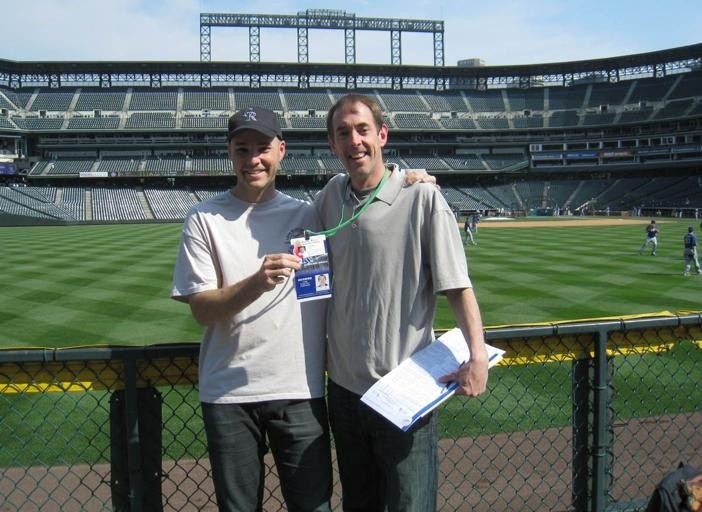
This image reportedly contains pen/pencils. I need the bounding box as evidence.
[441,360,465,393]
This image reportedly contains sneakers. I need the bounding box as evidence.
[637,252,656,256]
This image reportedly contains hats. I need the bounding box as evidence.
[227,106,282,139]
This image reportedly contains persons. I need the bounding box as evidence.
[296,246,306,260]
[443,189,515,233]
[518,197,702,221]
[636,221,660,257]
[170,104,443,512]
[683,227,702,277]
[315,275,328,288]
[463,217,478,247]
[309,91,490,512]
